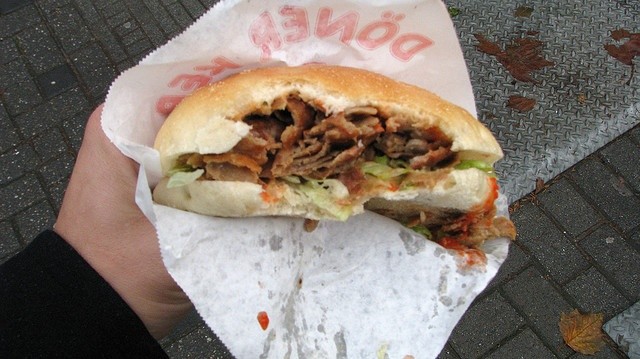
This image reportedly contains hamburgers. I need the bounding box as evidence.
[151,64,504,221]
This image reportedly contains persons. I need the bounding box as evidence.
[0,102,194,359]
